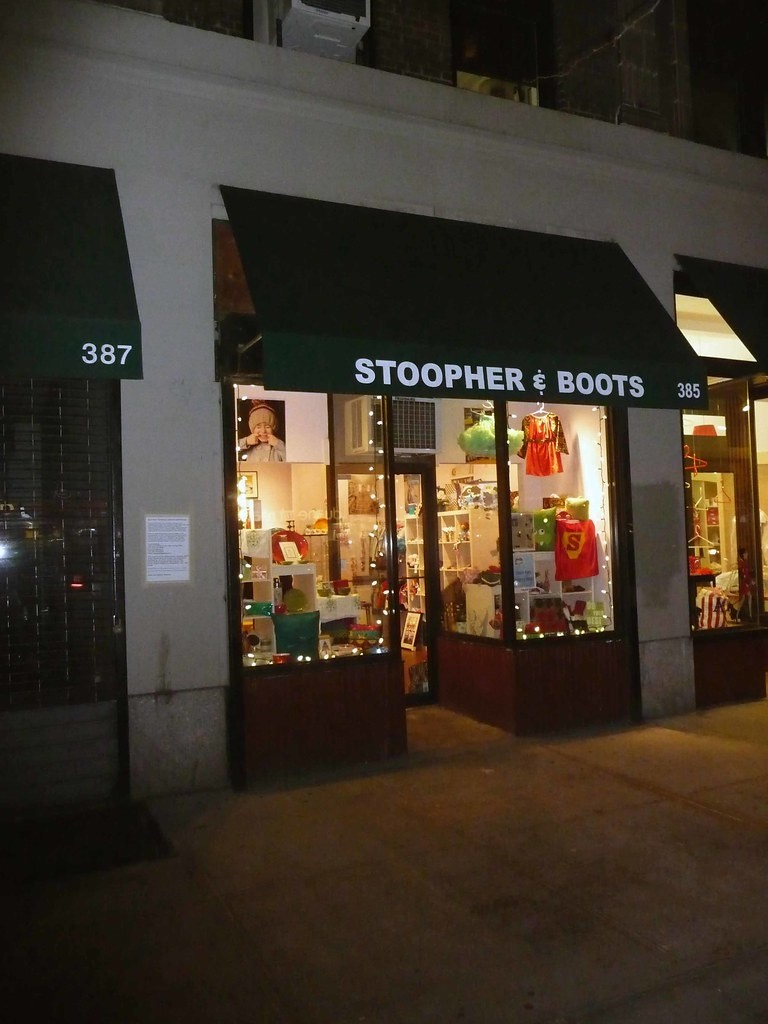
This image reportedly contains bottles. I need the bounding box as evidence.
[246,507,251,531]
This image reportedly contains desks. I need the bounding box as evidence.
[272,564,318,613]
[689,576,717,614]
[315,595,359,626]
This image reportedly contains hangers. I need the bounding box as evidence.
[688,525,715,548]
[530,401,549,416]
[694,486,709,510]
[713,479,732,503]
[682,445,707,471]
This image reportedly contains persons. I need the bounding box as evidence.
[238,400,286,462]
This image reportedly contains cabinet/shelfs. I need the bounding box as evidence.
[530,551,596,619]
[405,508,499,613]
[238,528,278,655]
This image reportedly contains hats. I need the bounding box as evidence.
[248,399,279,435]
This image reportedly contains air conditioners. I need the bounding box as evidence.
[346,391,441,456]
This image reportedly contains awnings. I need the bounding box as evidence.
[219,184,709,409]
[0,155,142,379]
[674,253,768,373]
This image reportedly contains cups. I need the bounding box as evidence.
[408,504,416,515]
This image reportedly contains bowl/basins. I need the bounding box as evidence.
[317,579,353,597]
[273,654,292,663]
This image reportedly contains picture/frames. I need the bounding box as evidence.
[236,470,258,499]
[400,610,424,651]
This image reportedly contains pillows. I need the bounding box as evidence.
[512,507,556,549]
[270,610,320,660]
